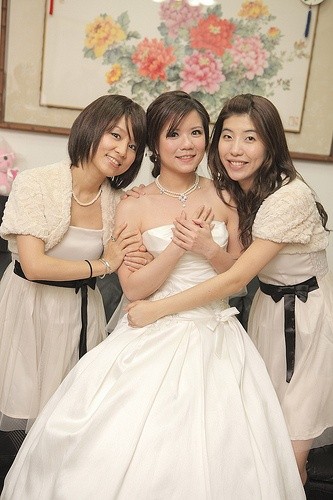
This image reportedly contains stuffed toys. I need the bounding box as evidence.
[0,148,21,196]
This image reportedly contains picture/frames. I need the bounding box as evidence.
[0,0,332,163]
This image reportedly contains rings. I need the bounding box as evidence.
[144,259,148,265]
[111,236,116,241]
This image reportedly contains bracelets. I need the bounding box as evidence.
[85,260,92,280]
[99,259,107,280]
[100,257,111,275]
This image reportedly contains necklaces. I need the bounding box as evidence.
[72,186,102,207]
[155,172,200,207]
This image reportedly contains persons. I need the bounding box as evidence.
[0,94,154,451]
[120,93,333,500]
[0,90,307,500]
